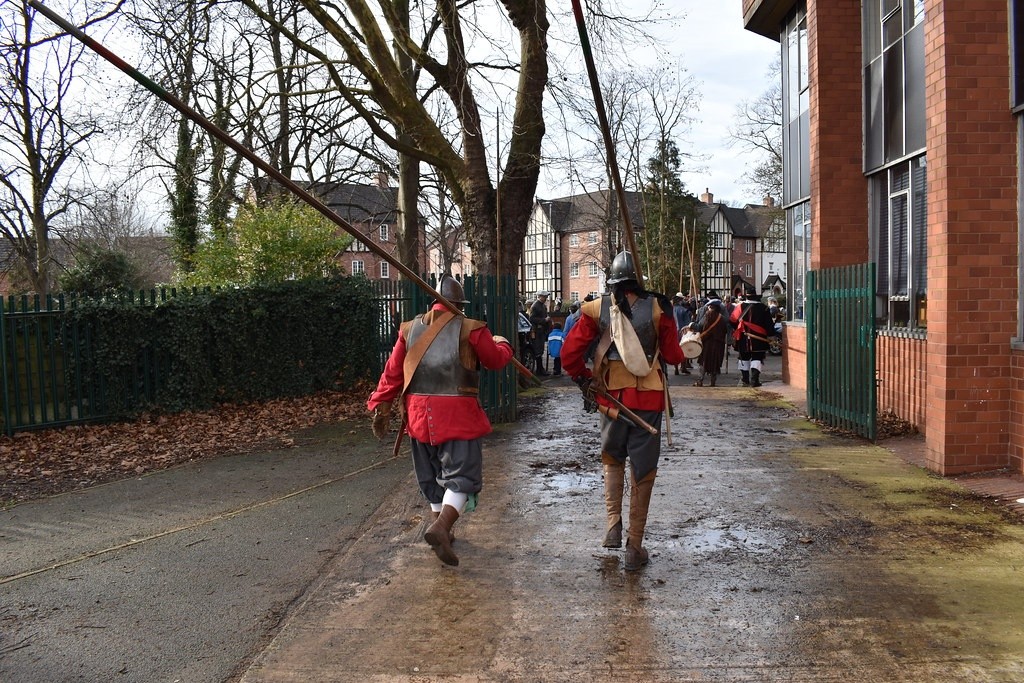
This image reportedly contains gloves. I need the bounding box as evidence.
[493,335,511,344]
[373,401,392,440]
[581,377,598,414]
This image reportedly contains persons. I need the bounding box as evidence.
[365,272,514,568]
[389,283,786,387]
[557,245,684,572]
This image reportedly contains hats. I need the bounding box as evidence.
[704,299,721,307]
[524,300,533,304]
[709,296,721,300]
[536,289,551,296]
[584,294,593,301]
[676,292,684,298]
[672,296,683,302]
[706,291,717,297]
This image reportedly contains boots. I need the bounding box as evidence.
[431,512,455,550]
[425,504,459,567]
[751,368,762,387]
[740,370,750,387]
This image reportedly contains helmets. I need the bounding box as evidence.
[431,272,471,306]
[607,250,648,284]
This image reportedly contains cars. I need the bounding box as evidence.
[461,306,537,375]
[764,321,783,357]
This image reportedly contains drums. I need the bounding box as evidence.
[679,326,704,359]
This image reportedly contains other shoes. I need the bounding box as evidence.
[604,516,622,548]
[674,359,715,386]
[626,544,648,570]
[536,368,563,378]
